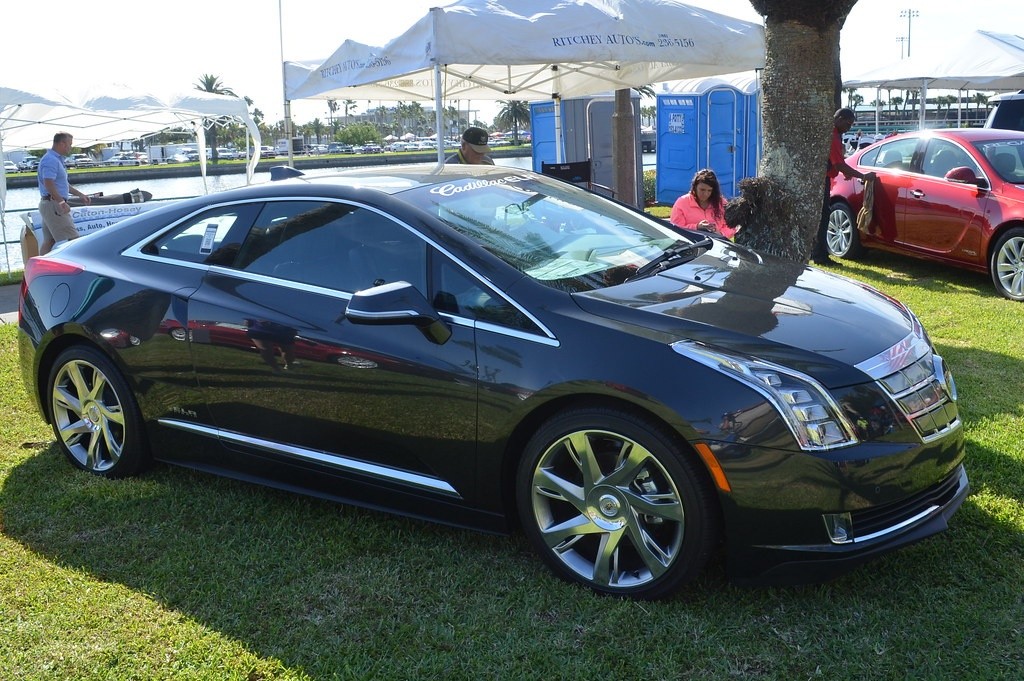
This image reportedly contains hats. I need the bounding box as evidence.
[462,128,491,153]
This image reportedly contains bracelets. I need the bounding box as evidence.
[862,174,865,182]
[58,199,65,205]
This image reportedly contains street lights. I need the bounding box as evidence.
[896,36,908,58]
[899,9,920,58]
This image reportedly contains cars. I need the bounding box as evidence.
[20,129,533,171]
[3,160,18,174]
[983,90,1024,132]
[17,161,967,594]
[822,127,1024,301]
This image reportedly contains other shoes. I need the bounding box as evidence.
[818,258,842,266]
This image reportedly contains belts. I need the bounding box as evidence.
[42,196,66,202]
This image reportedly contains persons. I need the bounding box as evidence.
[37,133,90,256]
[444,127,494,166]
[809,107,877,269]
[669,168,736,240]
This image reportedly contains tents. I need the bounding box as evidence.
[0,83,261,281]
[842,29,1024,130]
[281,0,767,169]
